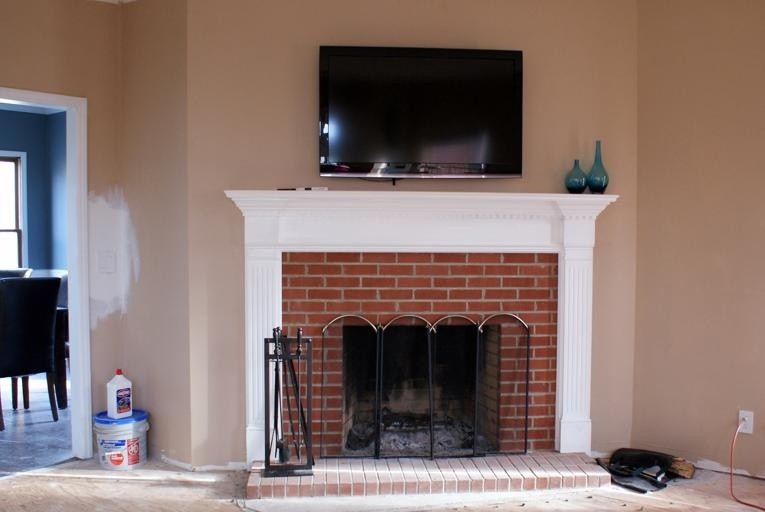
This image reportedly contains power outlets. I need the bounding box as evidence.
[737,410,754,435]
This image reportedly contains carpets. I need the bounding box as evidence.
[1,367,74,478]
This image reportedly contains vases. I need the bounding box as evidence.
[565,139,608,194]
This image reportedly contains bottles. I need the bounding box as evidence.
[566,141,608,192]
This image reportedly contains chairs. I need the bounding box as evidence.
[0,267,70,424]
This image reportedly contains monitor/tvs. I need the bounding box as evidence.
[319,46,523,178]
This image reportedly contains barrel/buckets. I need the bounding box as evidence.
[93,409,150,470]
